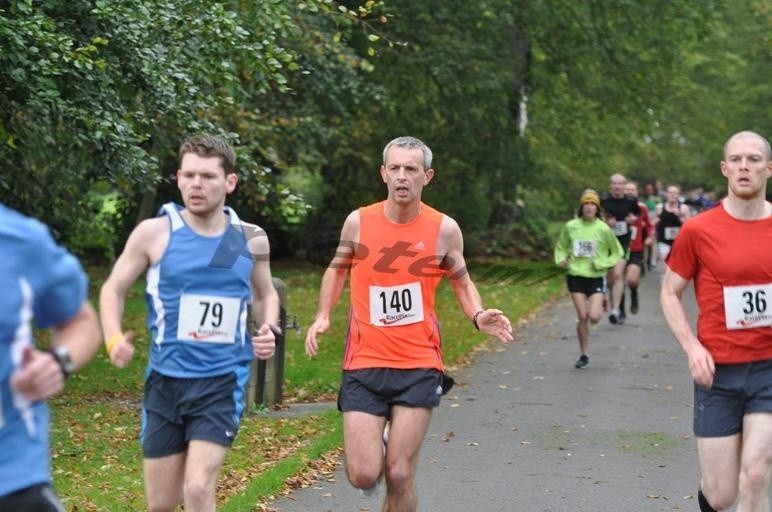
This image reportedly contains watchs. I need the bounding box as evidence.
[48,347,75,376]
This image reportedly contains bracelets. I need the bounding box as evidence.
[269,324,281,345]
[473,310,482,330]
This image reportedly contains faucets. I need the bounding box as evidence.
[286,315,301,335]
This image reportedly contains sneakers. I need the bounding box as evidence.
[574,355,591,368]
[609,291,639,325]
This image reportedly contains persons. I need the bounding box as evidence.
[1,201,104,511]
[97,133,279,512]
[552,170,719,370]
[660,131,772,512]
[306,137,514,512]
